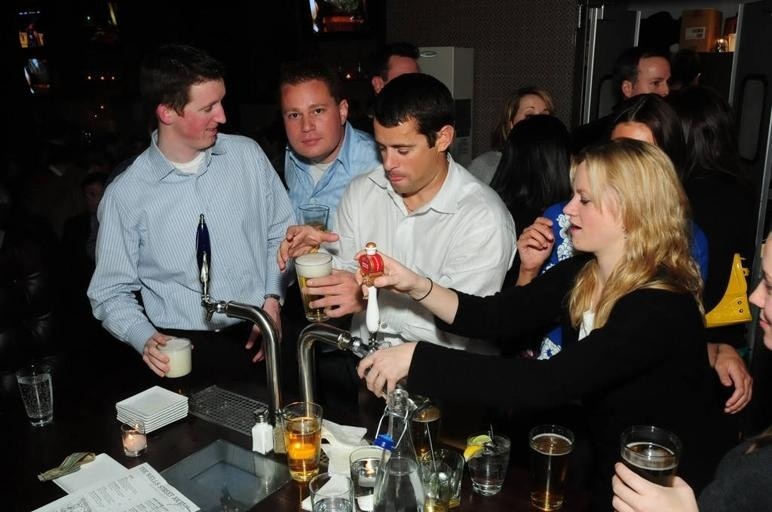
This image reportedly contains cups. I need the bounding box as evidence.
[14,364,54,427]
[296,202,330,236]
[155,337,193,382]
[281,401,323,483]
[621,424,682,485]
[529,424,577,510]
[468,432,512,497]
[120,419,148,459]
[421,448,465,508]
[293,250,333,323]
[382,452,427,512]
[308,472,354,512]
[348,446,392,510]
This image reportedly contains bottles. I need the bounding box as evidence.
[372,390,425,511]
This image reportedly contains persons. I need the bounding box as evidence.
[2,40,769,511]
